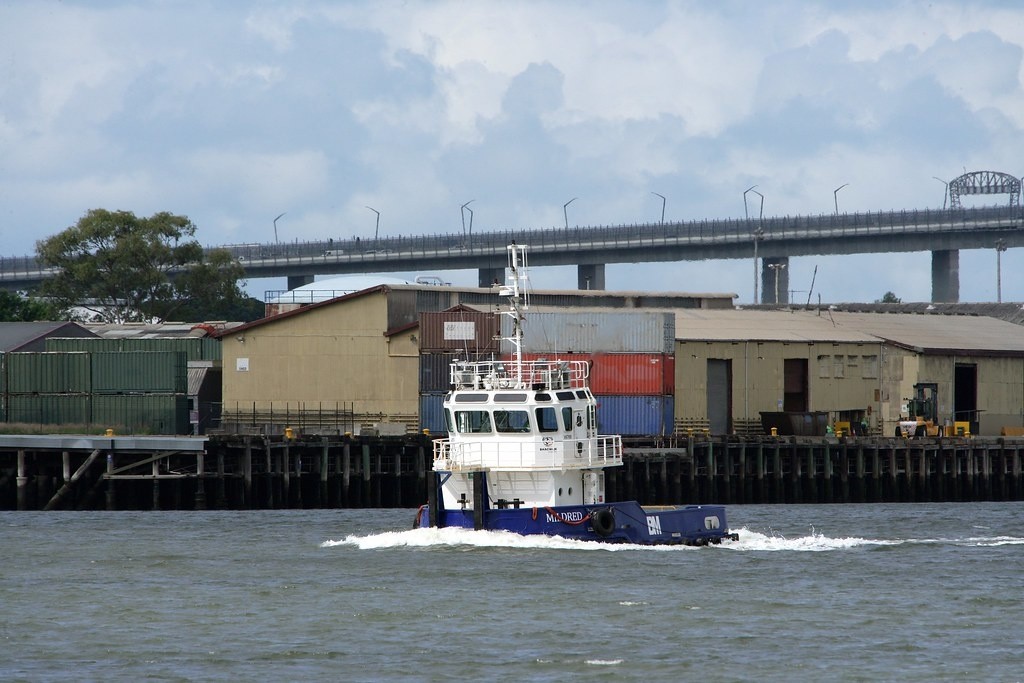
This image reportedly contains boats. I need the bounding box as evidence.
[412,239,741,548]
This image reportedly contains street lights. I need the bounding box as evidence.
[367,206,379,239]
[274,213,286,245]
[585,275,592,290]
[563,197,576,228]
[460,204,473,235]
[994,236,1007,304]
[932,176,949,210]
[743,185,758,219]
[768,264,787,303]
[650,191,665,225]
[750,189,764,219]
[461,200,472,235]
[752,226,766,305]
[834,184,848,215]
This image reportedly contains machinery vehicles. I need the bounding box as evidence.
[895,382,943,438]
